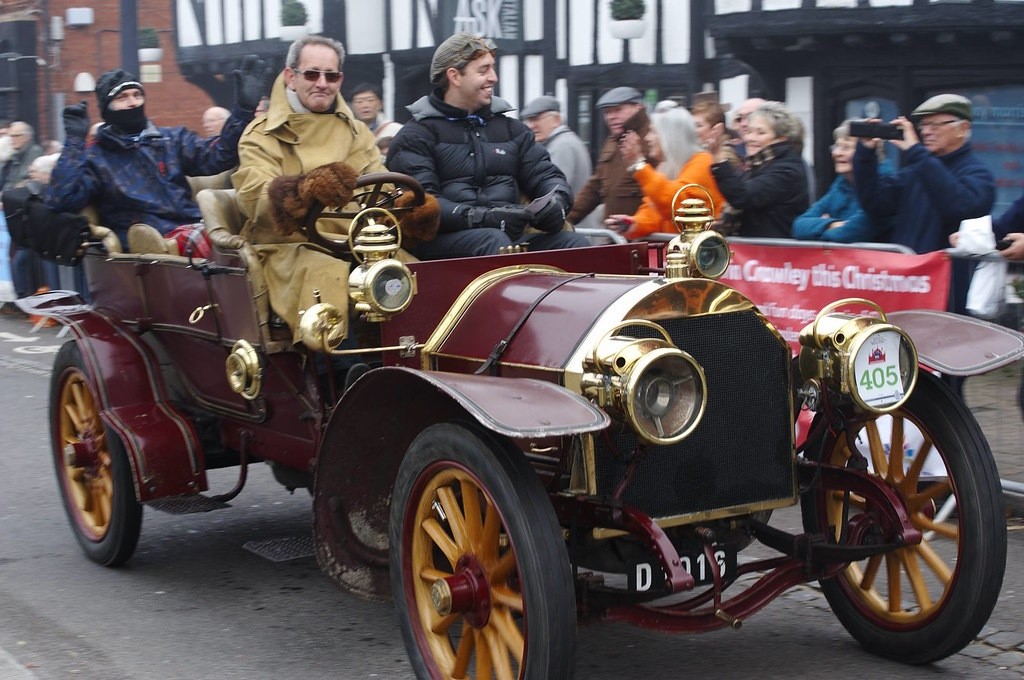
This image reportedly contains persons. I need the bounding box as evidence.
[568,84,658,243]
[791,116,895,240]
[852,92,997,253]
[385,33,590,260]
[231,34,441,400]
[949,194,1024,424]
[349,81,405,146]
[603,90,810,243]
[520,96,606,229]
[0,54,274,318]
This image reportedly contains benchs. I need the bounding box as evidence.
[197,190,628,354]
[0,168,234,254]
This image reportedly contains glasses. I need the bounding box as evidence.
[916,120,959,131]
[293,68,341,83]
[830,143,854,151]
[733,117,748,123]
[462,38,497,59]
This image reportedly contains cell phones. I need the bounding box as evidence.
[849,121,904,141]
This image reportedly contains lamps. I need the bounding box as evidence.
[74,70,96,93]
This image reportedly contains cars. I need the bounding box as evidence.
[0,170,1023,680]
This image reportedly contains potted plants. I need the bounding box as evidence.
[280,1,310,41]
[136,25,163,85]
[610,1,646,41]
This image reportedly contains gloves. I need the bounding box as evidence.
[233,55,273,109]
[268,161,360,235]
[473,205,529,241]
[62,98,92,138]
[528,194,568,233]
[377,190,439,240]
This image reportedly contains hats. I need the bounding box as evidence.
[490,96,516,114]
[594,86,641,108]
[519,97,561,118]
[95,69,142,108]
[911,94,972,121]
[430,34,489,83]
